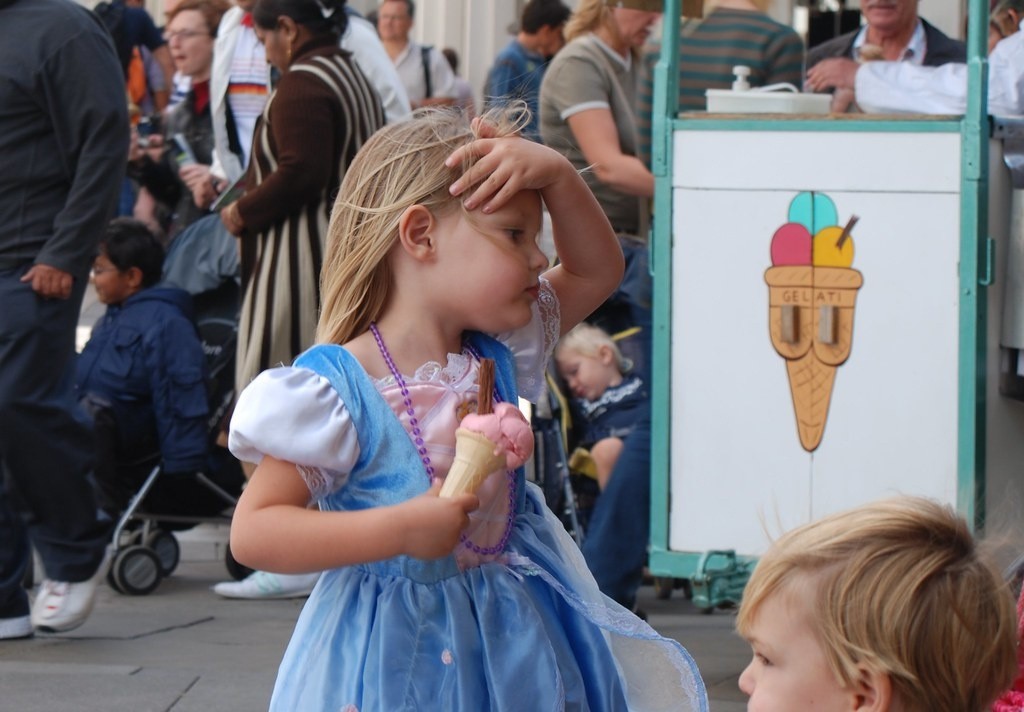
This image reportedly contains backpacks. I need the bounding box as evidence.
[93,1,124,62]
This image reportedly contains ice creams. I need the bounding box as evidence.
[439,403,535,498]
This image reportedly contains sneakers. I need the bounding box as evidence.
[0,617,32,638]
[216,572,324,598]
[32,543,113,631]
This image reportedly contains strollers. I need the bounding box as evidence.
[105,212,256,597]
[530,235,694,623]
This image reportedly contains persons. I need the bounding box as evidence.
[554,318,648,499]
[1,0,132,640]
[71,212,215,492]
[212,1,391,601]
[228,108,713,712]
[799,1,967,98]
[579,0,806,623]
[733,497,1016,712]
[803,2,1024,122]
[439,46,478,127]
[91,0,412,250]
[369,1,463,114]
[481,1,573,146]
[535,0,662,331]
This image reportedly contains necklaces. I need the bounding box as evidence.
[366,318,517,558]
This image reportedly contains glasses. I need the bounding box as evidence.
[162,28,211,42]
[92,266,120,277]
